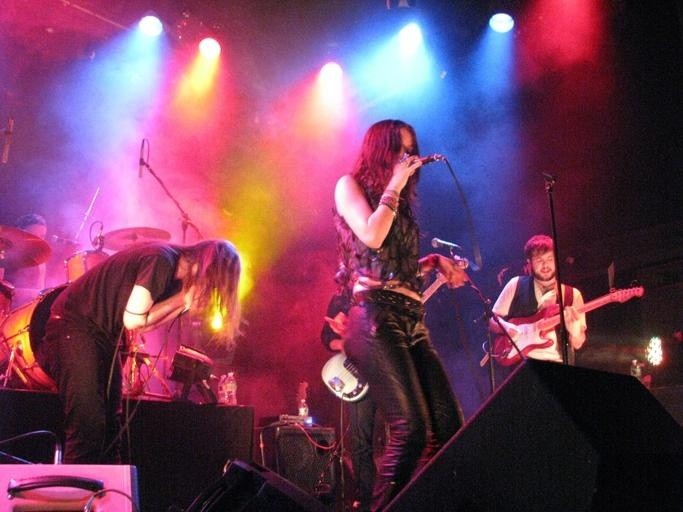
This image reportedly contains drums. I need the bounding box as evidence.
[1,278,15,317]
[0,281,130,394]
[64,248,109,284]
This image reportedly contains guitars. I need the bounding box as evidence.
[320,271,448,403]
[491,281,645,366]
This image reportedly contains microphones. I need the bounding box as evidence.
[93,225,104,248]
[1,116,15,164]
[430,237,464,253]
[406,151,442,170]
[137,136,147,177]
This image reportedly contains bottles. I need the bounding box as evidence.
[218,372,237,406]
[299,399,309,418]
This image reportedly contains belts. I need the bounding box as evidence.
[347,288,428,315]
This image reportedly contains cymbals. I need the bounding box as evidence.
[91,226,168,250]
[0,224,51,269]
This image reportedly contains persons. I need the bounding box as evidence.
[488,234,587,372]
[42,238,241,467]
[319,288,379,512]
[330,119,471,510]
[0,212,47,314]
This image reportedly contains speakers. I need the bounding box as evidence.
[377,354,683,512]
[186,455,330,512]
[260,425,337,498]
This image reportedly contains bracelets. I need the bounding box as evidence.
[378,189,401,215]
